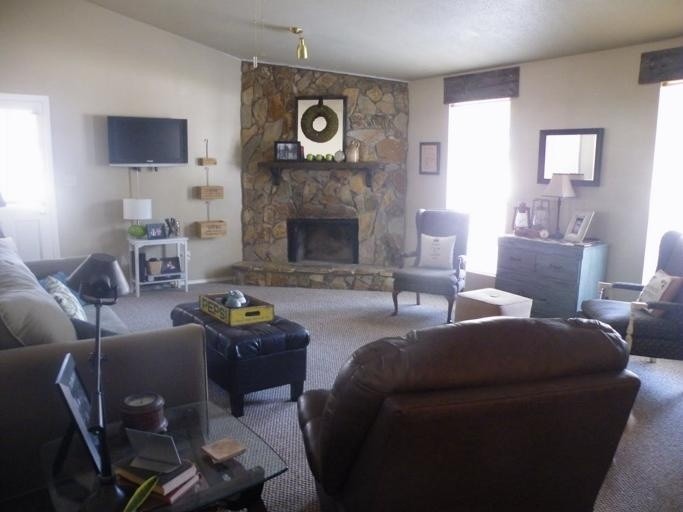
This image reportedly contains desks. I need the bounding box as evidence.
[128,238,188,298]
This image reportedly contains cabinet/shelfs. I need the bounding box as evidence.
[495,235,609,318]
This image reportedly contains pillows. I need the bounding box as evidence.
[0,237,78,347]
[636,269,683,318]
[418,233,456,269]
[39,276,88,323]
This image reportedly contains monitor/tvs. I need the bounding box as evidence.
[107,115,188,168]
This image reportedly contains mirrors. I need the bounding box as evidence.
[537,128,603,187]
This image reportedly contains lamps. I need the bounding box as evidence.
[291,26,307,61]
[122,198,152,238]
[541,173,576,240]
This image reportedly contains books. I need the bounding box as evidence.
[114,467,200,504]
[119,457,196,496]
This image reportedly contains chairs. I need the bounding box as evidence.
[297,317,641,512]
[576,230,683,362]
[392,208,470,324]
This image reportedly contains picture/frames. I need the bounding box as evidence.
[563,210,595,244]
[56,353,105,473]
[419,142,439,174]
[274,141,301,162]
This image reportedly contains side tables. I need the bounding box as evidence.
[39,401,288,511]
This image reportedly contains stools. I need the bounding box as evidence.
[455,288,533,324]
[170,302,310,418]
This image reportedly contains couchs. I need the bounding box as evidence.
[0,238,208,502]
